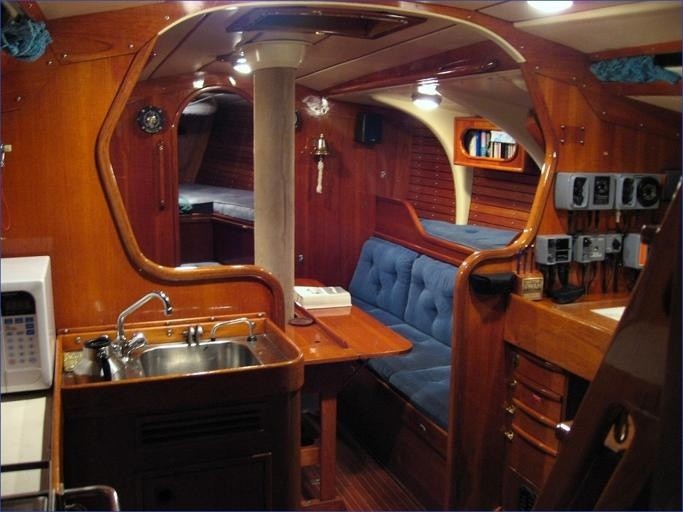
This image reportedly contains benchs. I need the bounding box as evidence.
[323,237,465,511]
[179,181,254,265]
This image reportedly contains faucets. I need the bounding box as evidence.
[114,291,173,359]
[210,316,255,342]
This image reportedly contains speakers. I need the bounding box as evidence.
[357,112,384,143]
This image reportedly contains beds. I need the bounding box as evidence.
[372,197,525,267]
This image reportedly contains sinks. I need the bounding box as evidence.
[140,342,263,377]
[590,306,626,322]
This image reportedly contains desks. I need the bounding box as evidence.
[289,272,416,502]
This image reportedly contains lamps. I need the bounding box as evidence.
[231,60,250,74]
[411,93,441,112]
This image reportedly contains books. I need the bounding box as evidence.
[294,285,352,310]
[463,129,515,161]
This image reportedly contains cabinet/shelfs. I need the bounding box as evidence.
[62,386,303,512]
[499,350,569,511]
[452,116,535,172]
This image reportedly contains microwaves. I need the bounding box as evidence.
[1,255,55,395]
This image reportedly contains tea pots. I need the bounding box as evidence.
[72,337,126,383]
